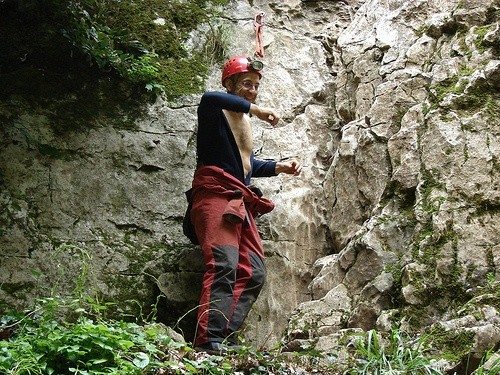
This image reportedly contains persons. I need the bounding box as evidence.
[191,55,303,355]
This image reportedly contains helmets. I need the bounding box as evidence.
[221,56,262,81]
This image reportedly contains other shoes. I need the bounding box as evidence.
[203,342,243,356]
